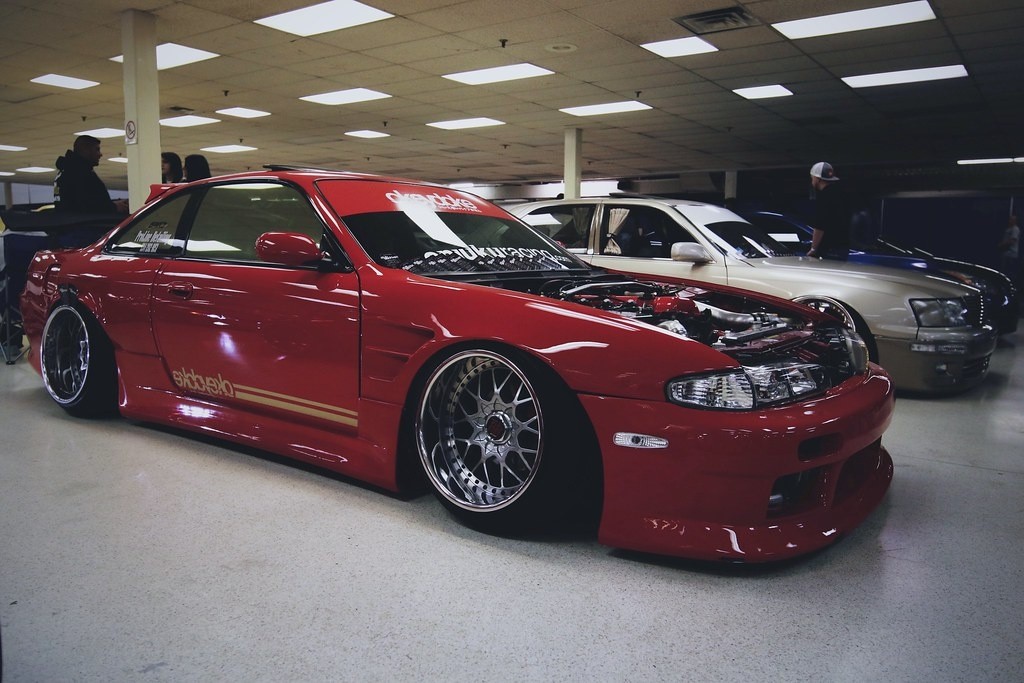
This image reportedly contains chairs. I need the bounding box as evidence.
[368,212,421,264]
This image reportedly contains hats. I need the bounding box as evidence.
[810,161,840,181]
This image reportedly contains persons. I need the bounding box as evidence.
[998,215,1019,276]
[184,154,210,180]
[161,152,183,184]
[54,135,118,246]
[807,162,849,261]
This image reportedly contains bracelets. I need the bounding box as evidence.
[811,248,815,251]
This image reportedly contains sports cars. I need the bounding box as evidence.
[461,197,997,399]
[18,164,895,567]
[620,207,1021,337]
